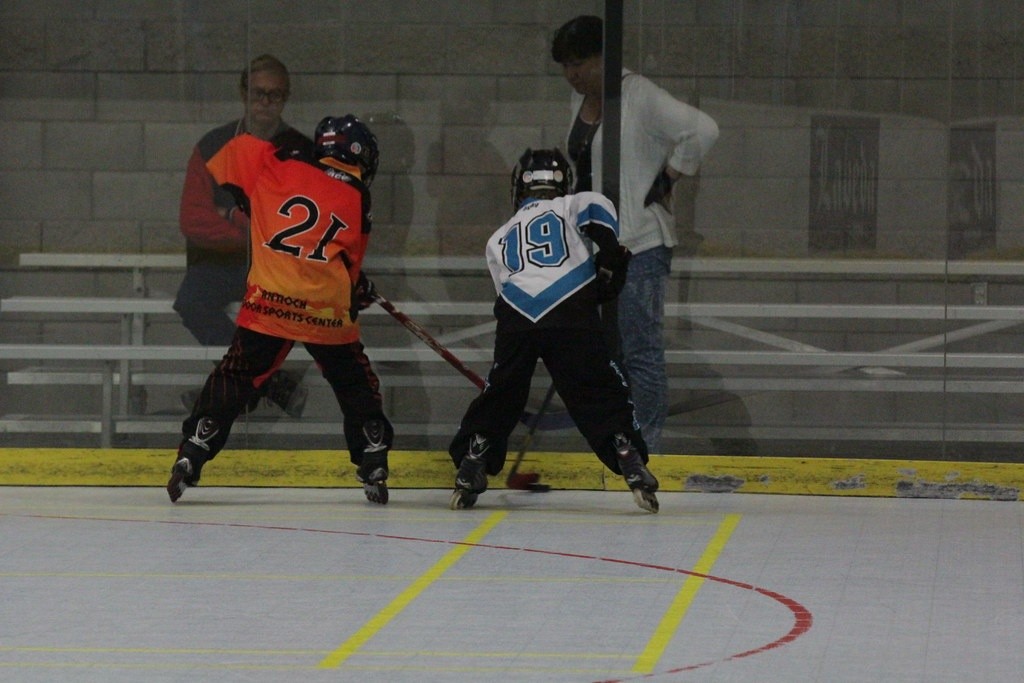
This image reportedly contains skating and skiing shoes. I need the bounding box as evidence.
[448,432,489,510]
[355,422,388,506]
[165,416,217,503]
[613,431,659,514]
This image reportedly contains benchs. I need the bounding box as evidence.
[0,255,1024,451]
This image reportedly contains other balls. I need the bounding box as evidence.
[527,483,552,494]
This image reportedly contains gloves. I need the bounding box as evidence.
[643,166,676,209]
[350,267,378,311]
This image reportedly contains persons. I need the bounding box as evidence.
[448,147,660,514]
[169,53,319,418]
[167,113,394,504]
[360,102,514,450]
[552,14,721,451]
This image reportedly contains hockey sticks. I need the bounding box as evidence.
[506,382,557,490]
[368,290,578,432]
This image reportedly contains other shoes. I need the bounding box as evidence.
[637,428,661,453]
[275,367,307,417]
[178,388,204,414]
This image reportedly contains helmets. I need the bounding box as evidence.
[511,145,574,214]
[313,114,378,177]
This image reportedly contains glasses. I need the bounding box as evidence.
[245,87,287,105]
